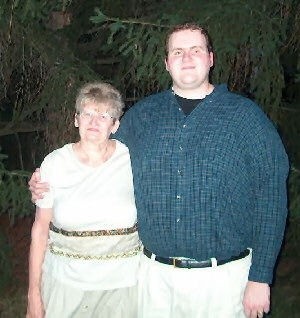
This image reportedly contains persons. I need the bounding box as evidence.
[27,81,143,318]
[28,23,290,317]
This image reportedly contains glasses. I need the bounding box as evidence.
[79,109,114,122]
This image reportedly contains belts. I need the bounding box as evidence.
[141,248,250,269]
